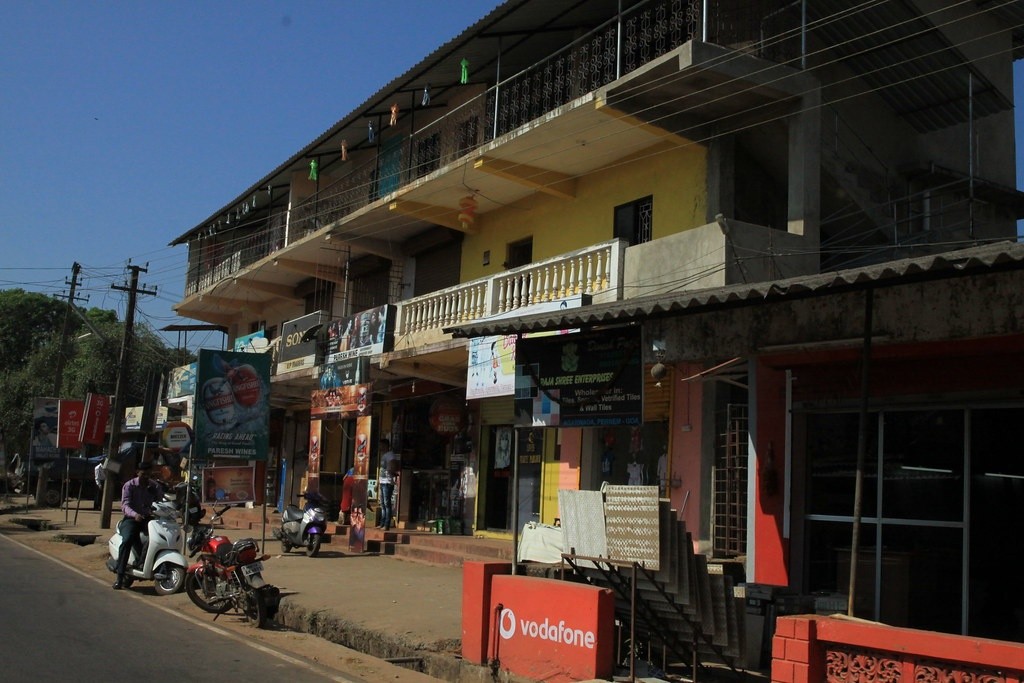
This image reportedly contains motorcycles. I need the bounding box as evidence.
[182,488,281,628]
[104,500,188,596]
[271,489,337,557]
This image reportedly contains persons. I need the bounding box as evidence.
[328,306,385,353]
[374,438,396,531]
[326,390,341,406]
[321,365,341,389]
[113,462,163,590]
[349,504,365,547]
[94,458,106,510]
[33,422,57,447]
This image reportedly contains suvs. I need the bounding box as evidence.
[26,441,165,511]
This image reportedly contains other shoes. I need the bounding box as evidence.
[375,525,383,529]
[113,581,123,590]
[94,506,101,511]
[381,526,389,531]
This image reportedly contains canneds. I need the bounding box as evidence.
[357,387,367,411]
[310,435,318,459]
[357,433,367,459]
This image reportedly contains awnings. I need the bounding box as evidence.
[436,242,1024,336]
